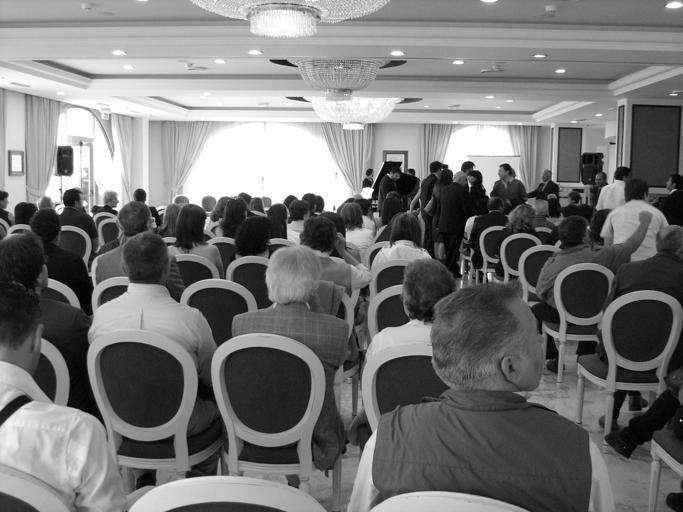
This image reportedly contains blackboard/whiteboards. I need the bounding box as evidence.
[467,154,520,200]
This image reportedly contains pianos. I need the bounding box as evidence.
[359,160,416,210]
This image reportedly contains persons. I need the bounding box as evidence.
[361,161,683,511]
[0,187,617,511]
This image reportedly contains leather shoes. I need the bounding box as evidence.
[628,394,649,412]
[598,415,619,430]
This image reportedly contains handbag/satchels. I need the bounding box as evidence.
[424,196,436,217]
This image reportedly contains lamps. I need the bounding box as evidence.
[191,0,405,131]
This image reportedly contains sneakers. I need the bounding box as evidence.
[665,493,682,511]
[546,361,558,373]
[604,432,634,459]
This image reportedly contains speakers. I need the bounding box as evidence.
[580,153,603,185]
[56,145,74,176]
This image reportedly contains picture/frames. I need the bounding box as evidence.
[382,149,408,173]
[8,150,24,176]
